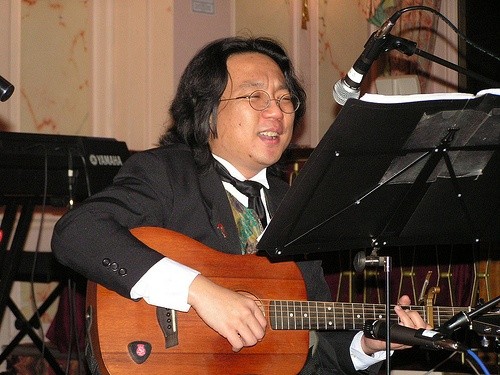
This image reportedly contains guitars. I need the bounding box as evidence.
[86,227,500,375]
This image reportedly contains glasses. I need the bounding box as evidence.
[218,90,301,115]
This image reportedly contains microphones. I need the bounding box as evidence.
[332,11,401,105]
[363,319,466,352]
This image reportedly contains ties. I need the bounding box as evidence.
[212,157,268,232]
